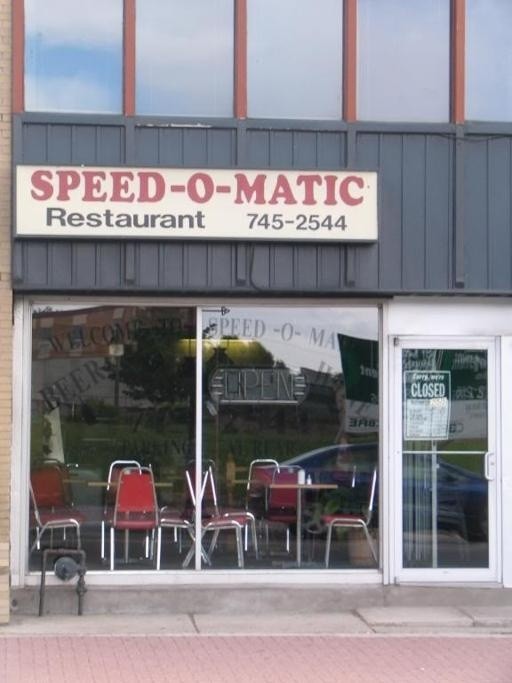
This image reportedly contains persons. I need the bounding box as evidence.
[331,372,352,445]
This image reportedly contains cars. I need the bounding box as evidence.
[245,436,489,546]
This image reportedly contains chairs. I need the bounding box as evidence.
[31,459,85,562]
[184,456,339,569]
[88,457,174,569]
[311,463,378,568]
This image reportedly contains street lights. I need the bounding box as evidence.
[107,329,125,413]
